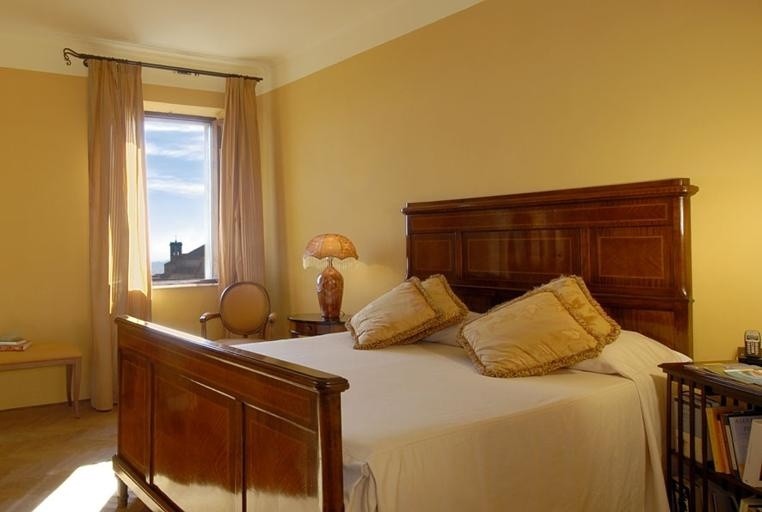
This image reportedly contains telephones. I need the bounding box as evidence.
[737,330,762,366]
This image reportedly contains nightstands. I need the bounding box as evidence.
[287,313,348,341]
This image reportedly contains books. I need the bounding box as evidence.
[684,361,762,387]
[668,381,762,511]
[0,340,33,351]
[0,337,26,346]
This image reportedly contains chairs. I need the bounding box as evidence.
[200,282,278,346]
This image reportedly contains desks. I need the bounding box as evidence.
[1,341,84,418]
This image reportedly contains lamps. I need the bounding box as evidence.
[301,233,358,320]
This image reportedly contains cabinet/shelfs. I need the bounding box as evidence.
[656,360,761,512]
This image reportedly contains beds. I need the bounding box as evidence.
[113,178,699,512]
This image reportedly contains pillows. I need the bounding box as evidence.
[534,273,621,347]
[347,276,444,352]
[390,274,469,346]
[455,286,605,377]
